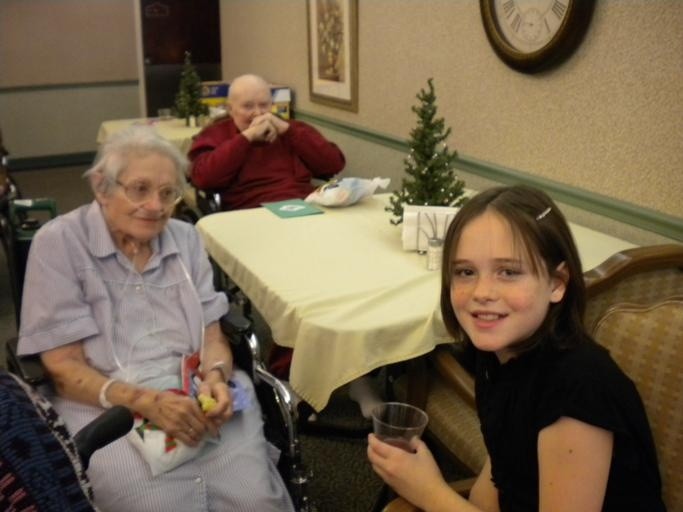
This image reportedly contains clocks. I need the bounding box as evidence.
[479,0,596,74]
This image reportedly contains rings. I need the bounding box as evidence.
[186,428,194,434]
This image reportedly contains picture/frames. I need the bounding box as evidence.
[305,0,358,115]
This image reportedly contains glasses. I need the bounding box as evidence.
[114,179,183,208]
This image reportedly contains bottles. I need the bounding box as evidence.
[426,237,443,271]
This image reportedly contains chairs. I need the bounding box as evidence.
[380,295,683,511]
[371,241,683,474]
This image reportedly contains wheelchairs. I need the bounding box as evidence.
[6,289,319,510]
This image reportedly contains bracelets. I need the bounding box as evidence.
[98,378,118,409]
[207,360,229,383]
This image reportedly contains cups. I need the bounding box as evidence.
[158,108,171,122]
[370,402,429,453]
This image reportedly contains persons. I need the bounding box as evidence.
[365,182,669,512]
[187,74,386,422]
[17,122,298,512]
